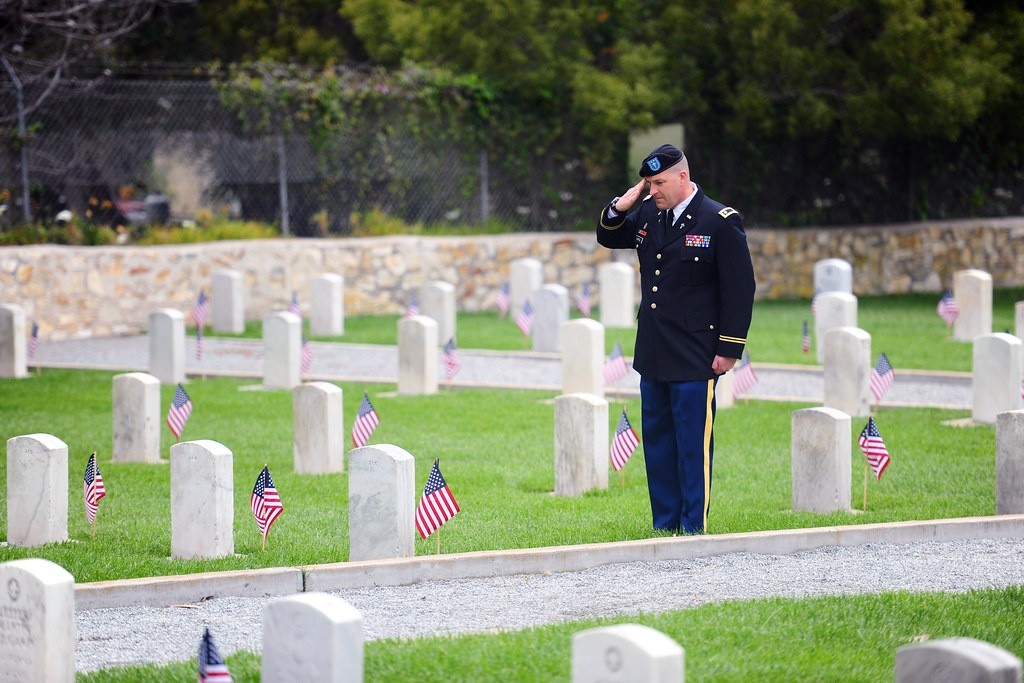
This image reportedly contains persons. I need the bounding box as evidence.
[0,180,170,237]
[597,145,757,537]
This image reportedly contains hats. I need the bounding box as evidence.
[639,144,683,177]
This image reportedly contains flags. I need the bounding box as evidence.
[197,632,235,683]
[415,460,460,541]
[27,322,41,359]
[248,465,283,555]
[191,278,961,406]
[855,418,891,482]
[351,396,379,449]
[609,408,639,472]
[165,383,193,440]
[83,454,105,524]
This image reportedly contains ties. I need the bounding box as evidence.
[666,208,675,239]
